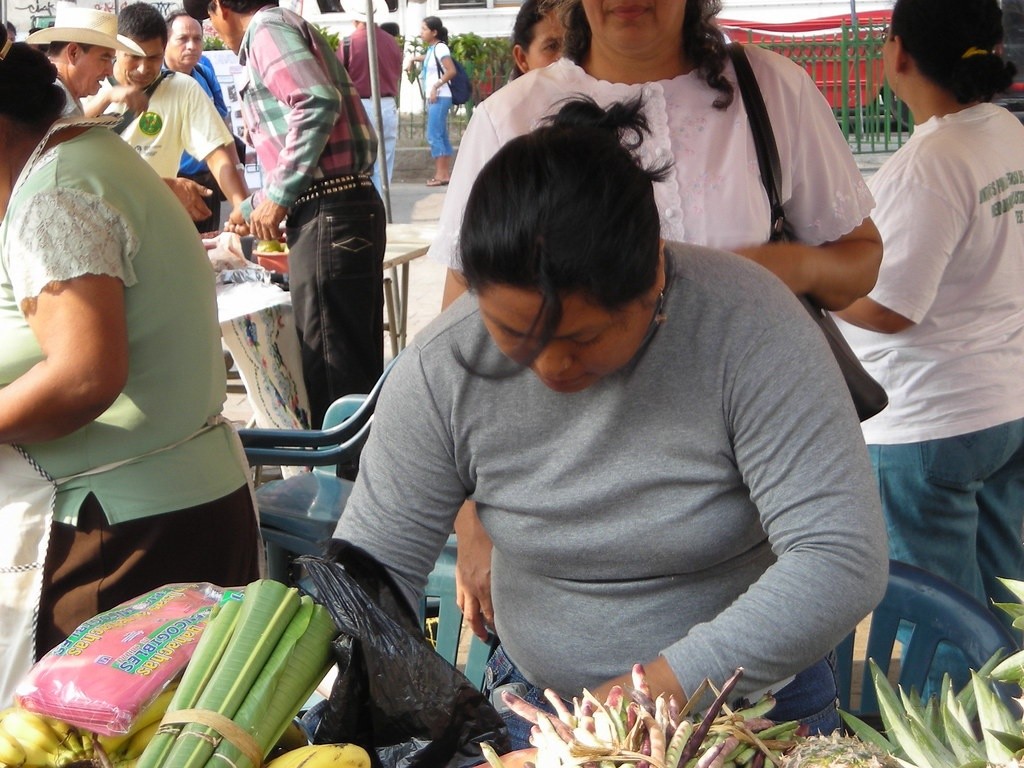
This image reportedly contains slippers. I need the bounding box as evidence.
[426,178,449,186]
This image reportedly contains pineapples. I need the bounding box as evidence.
[778,727,901,768]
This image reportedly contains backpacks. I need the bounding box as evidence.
[434,42,473,104]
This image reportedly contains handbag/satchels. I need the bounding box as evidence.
[287,555,511,767]
[771,220,889,423]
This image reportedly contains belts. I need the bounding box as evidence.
[290,175,372,210]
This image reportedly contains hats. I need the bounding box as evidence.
[182,0,215,21]
[340,0,390,23]
[23,6,147,58]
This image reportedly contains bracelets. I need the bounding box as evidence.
[432,79,444,89]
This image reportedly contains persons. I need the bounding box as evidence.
[426,0,885,643]
[332,103,891,753]
[0,22,263,663]
[507,0,575,83]
[830,0,1024,711]
[24,0,402,482]
[406,16,457,186]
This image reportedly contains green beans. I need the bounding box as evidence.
[479,664,799,768]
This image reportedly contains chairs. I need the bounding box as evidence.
[820,561,1016,718]
[239,348,496,697]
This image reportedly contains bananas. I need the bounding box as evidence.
[261,716,371,768]
[0,682,178,768]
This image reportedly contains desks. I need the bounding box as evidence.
[198,226,435,357]
